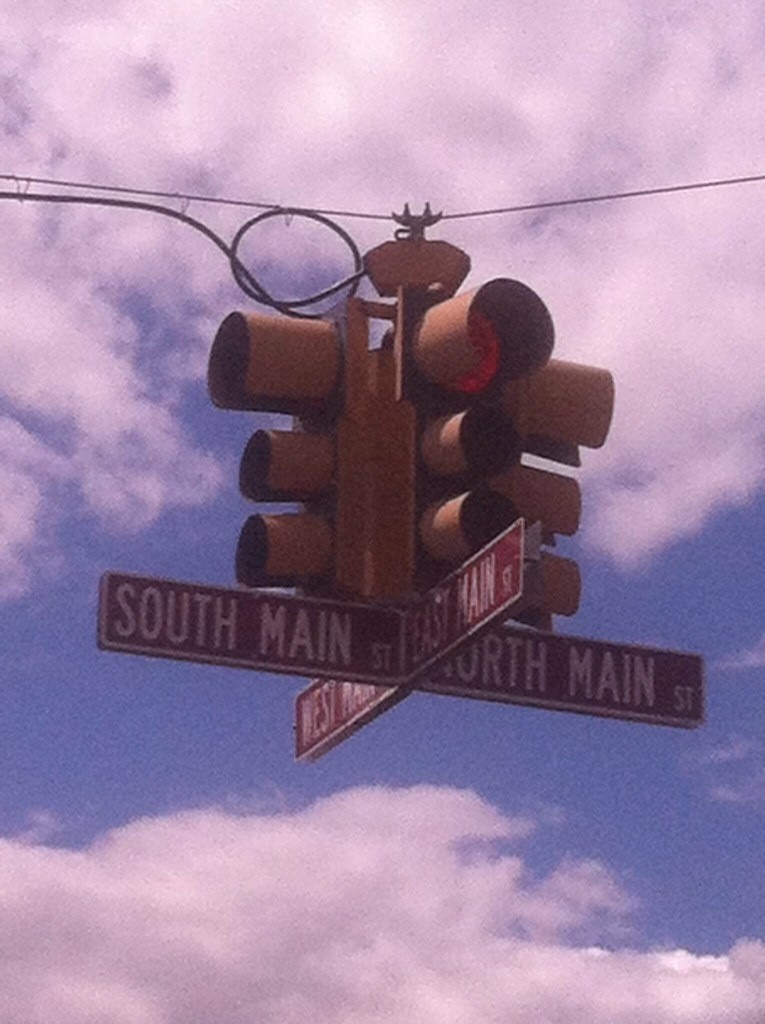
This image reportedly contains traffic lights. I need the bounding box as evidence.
[500,352,614,624]
[209,296,373,600]
[393,280,557,621]
